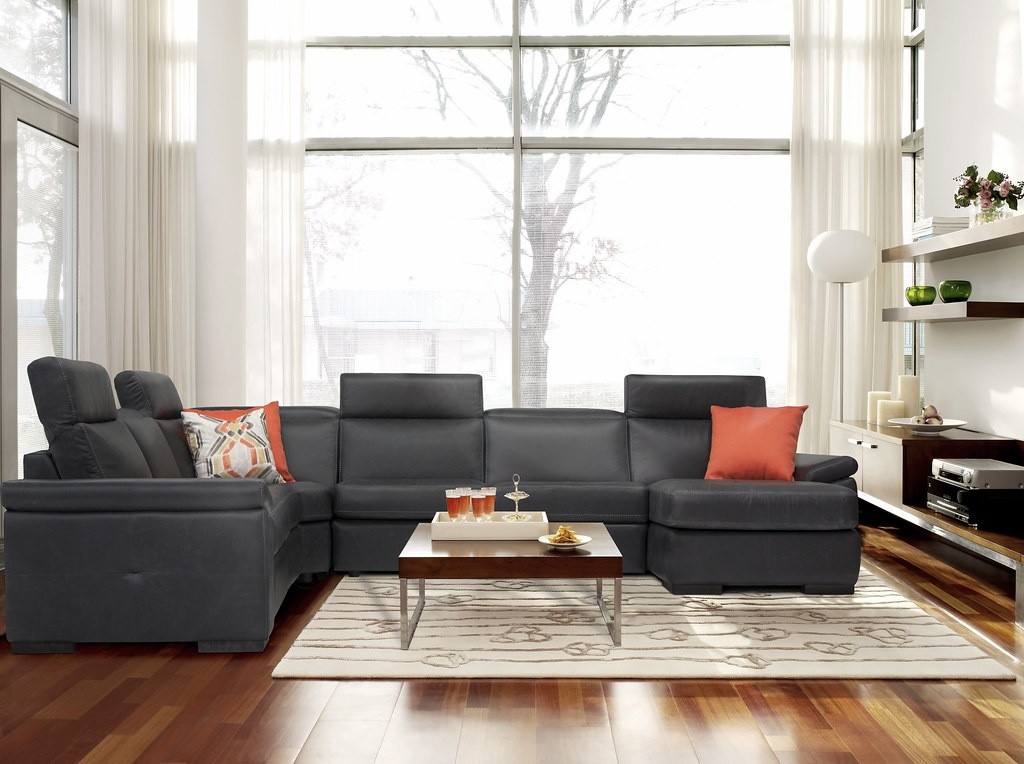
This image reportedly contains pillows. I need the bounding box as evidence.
[182,400,296,483]
[180,408,286,484]
[704,405,808,482]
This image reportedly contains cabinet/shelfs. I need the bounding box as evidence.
[828,420,903,516]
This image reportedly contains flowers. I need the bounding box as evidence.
[953,160,1024,216]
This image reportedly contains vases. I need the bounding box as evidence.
[905,286,937,306]
[968,210,1013,230]
[938,280,972,304]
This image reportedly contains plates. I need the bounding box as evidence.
[888,418,968,437]
[538,535,592,553]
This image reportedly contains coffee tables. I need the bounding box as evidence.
[398,522,623,650]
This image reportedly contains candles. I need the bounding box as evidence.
[876,399,905,427]
[897,374,921,419]
[866,390,892,425]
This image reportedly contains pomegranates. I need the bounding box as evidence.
[910,404,943,426]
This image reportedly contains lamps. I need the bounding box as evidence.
[806,229,877,424]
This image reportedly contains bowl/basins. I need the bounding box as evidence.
[938,280,972,303]
[905,286,937,306]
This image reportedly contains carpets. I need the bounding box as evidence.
[271,565,1017,680]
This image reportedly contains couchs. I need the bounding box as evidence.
[1,356,863,654]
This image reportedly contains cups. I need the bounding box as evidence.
[469,489,486,522]
[445,490,461,522]
[455,487,472,522]
[481,487,497,522]
